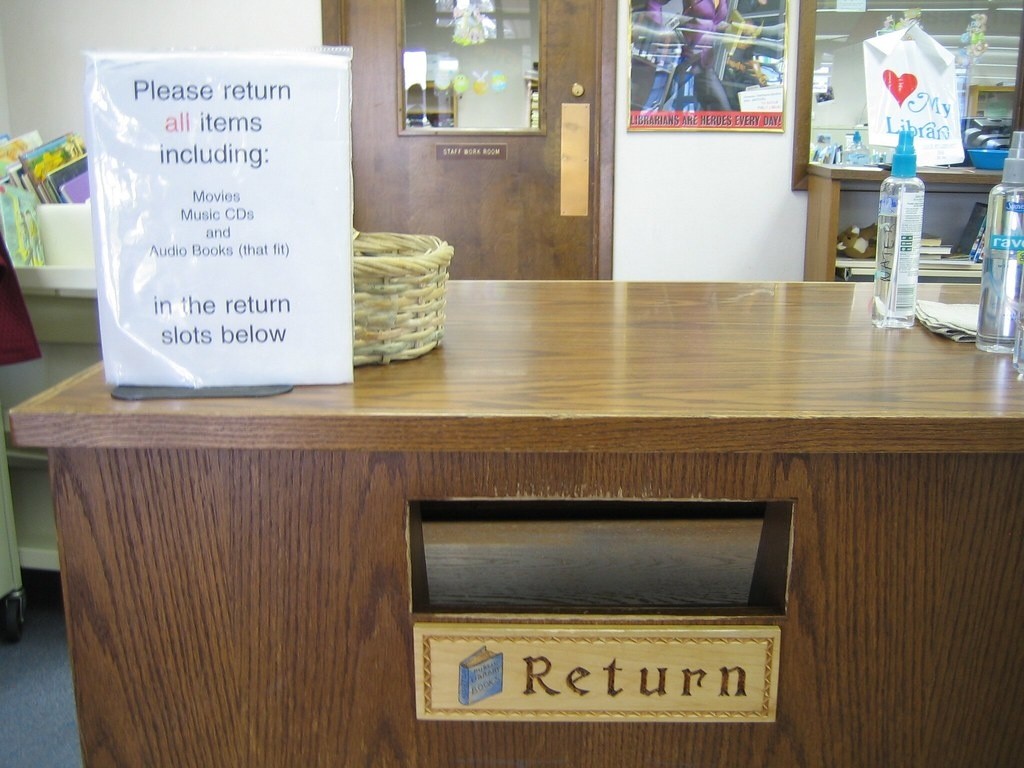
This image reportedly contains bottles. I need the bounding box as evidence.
[974,130,1023,355]
[813,131,887,165]
[869,130,926,330]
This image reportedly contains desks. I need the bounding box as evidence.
[802,161,1005,274]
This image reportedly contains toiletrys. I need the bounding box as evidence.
[870,130,925,330]
[974,130,1024,356]
[812,131,887,164]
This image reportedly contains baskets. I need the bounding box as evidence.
[351,231,454,366]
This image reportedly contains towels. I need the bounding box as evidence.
[915,299,979,342]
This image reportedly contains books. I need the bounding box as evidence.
[920,234,952,260]
[0,129,89,204]
[955,202,989,263]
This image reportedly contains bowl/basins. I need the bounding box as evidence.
[966,148,1009,171]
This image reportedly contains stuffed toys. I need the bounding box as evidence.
[837,223,878,259]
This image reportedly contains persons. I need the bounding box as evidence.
[633,0,769,112]
